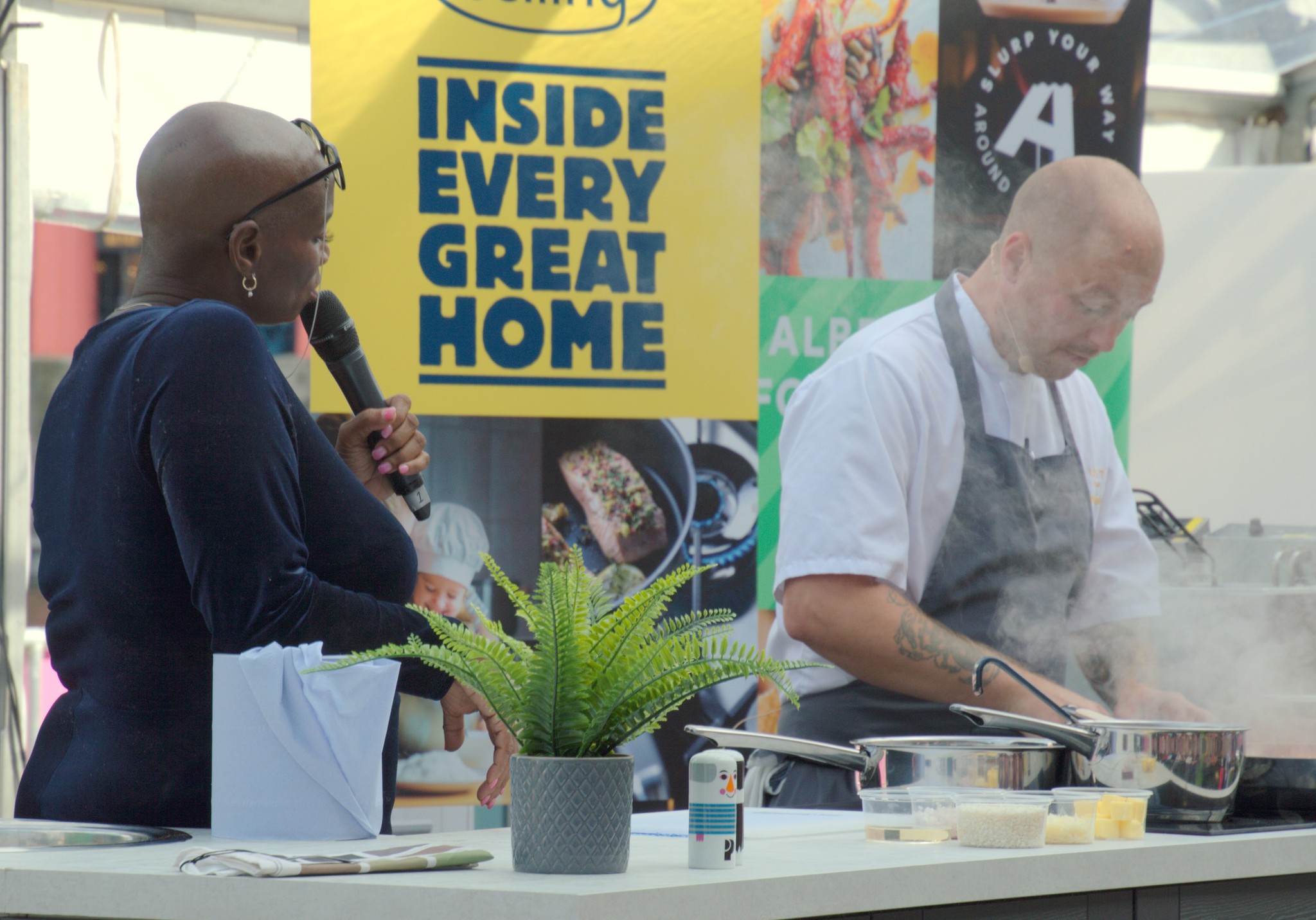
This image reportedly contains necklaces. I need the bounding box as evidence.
[111,298,154,315]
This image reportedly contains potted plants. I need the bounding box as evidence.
[305,541,835,875]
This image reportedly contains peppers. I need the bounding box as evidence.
[758,1,937,285]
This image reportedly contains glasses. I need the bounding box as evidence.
[230,117,348,236]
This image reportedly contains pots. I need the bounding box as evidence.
[684,723,1069,796]
[950,702,1251,824]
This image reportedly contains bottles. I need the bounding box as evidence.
[687,750,747,871]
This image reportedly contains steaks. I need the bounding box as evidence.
[558,443,670,568]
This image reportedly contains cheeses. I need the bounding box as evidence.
[1073,794,1147,840]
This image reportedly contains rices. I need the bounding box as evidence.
[956,803,1047,849]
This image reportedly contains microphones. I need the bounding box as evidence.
[298,289,432,524]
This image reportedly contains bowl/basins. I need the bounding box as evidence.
[856,784,1157,852]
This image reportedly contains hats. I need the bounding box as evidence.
[410,501,492,589]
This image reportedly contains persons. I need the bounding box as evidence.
[737,154,1164,814]
[392,499,490,772]
[16,105,514,830]
